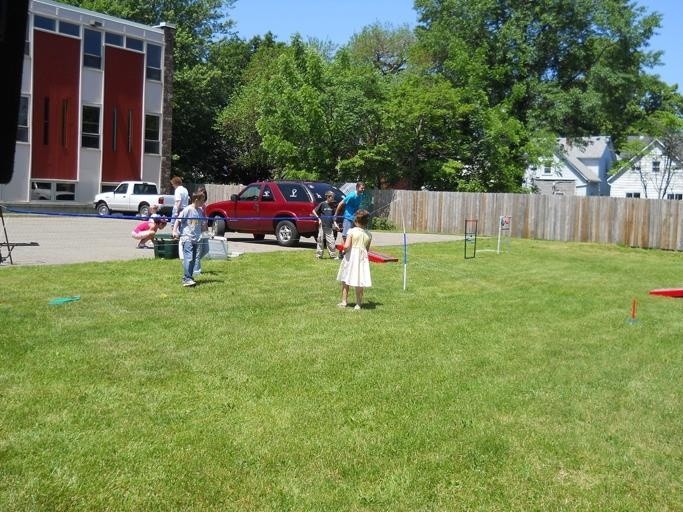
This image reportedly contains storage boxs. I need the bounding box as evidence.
[151,233,179,259]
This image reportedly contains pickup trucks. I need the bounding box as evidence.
[93,180,192,224]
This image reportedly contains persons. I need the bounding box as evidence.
[133,221,168,247]
[149,203,158,231]
[311,191,337,259]
[192,185,210,274]
[335,207,373,311]
[169,175,190,231]
[333,181,366,259]
[172,191,207,286]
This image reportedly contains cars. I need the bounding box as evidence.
[203,180,350,248]
[31,181,76,202]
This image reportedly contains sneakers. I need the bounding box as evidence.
[354,304,362,310]
[184,280,196,287]
[337,302,347,308]
